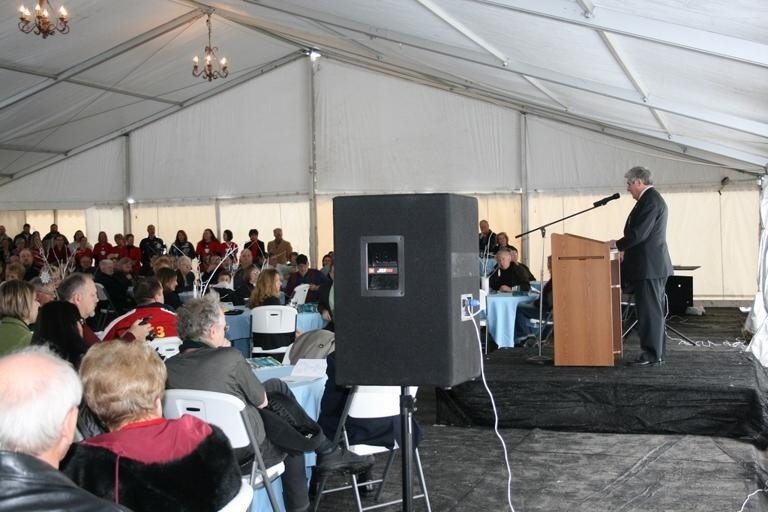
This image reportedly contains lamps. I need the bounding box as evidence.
[191,10,228,83]
[16,0,70,38]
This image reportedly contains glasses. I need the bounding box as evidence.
[224,324,229,332]
[78,317,86,325]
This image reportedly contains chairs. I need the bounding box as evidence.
[286,284,310,306]
[163,389,285,511]
[248,306,298,366]
[522,307,553,353]
[314,388,434,512]
[149,336,183,359]
[95,283,120,330]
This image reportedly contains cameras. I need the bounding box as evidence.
[138,315,156,343]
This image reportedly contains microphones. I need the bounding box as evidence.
[593,192,620,207]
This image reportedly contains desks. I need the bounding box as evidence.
[551,233,621,365]
[487,289,540,349]
[212,305,332,342]
[248,366,327,512]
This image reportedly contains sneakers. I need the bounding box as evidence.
[317,443,374,474]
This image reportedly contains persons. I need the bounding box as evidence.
[244,229,265,263]
[196,228,220,256]
[174,256,195,293]
[42,223,69,246]
[139,225,164,275]
[202,255,224,283]
[320,255,332,279]
[158,267,183,310]
[218,271,231,284]
[249,268,296,350]
[30,231,48,269]
[268,228,292,264]
[221,230,238,263]
[125,234,140,260]
[0,342,133,512]
[233,248,253,289]
[57,271,154,348]
[232,264,261,306]
[515,255,552,348]
[93,232,112,267]
[169,230,195,259]
[605,166,674,368]
[200,253,212,272]
[70,230,93,254]
[21,224,32,240]
[100,259,133,314]
[19,249,39,281]
[0,280,40,359]
[221,256,236,274]
[164,295,376,511]
[13,238,27,255]
[75,254,95,275]
[118,257,137,286]
[111,234,127,260]
[29,276,56,305]
[0,225,9,241]
[286,254,328,303]
[317,264,334,332]
[5,262,24,282]
[288,251,298,266]
[0,239,13,263]
[490,246,531,291]
[497,232,518,252]
[153,256,171,273]
[49,235,70,264]
[266,252,284,281]
[479,220,498,258]
[102,276,179,341]
[29,301,88,372]
[60,339,254,512]
[40,265,61,292]
[507,246,537,281]
[75,237,92,256]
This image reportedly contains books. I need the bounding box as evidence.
[279,359,328,383]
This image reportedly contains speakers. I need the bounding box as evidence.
[334,193,484,390]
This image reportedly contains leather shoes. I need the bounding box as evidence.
[627,357,661,367]
[310,482,325,501]
[357,479,375,496]
